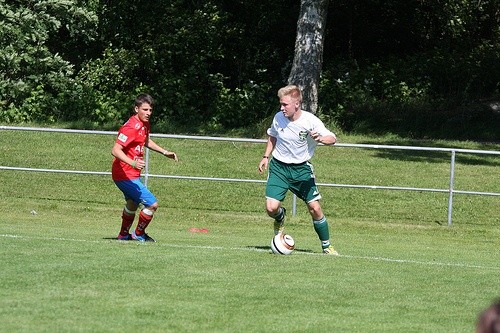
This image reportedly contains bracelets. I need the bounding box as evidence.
[262,156,269,158]
[163,151,167,155]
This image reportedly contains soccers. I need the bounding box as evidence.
[270,233,295,255]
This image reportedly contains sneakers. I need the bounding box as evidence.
[273,207,287,235]
[117,234,136,241]
[322,245,339,256]
[131,230,157,243]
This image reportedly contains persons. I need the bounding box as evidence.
[111,94,178,242]
[259,85,339,256]
